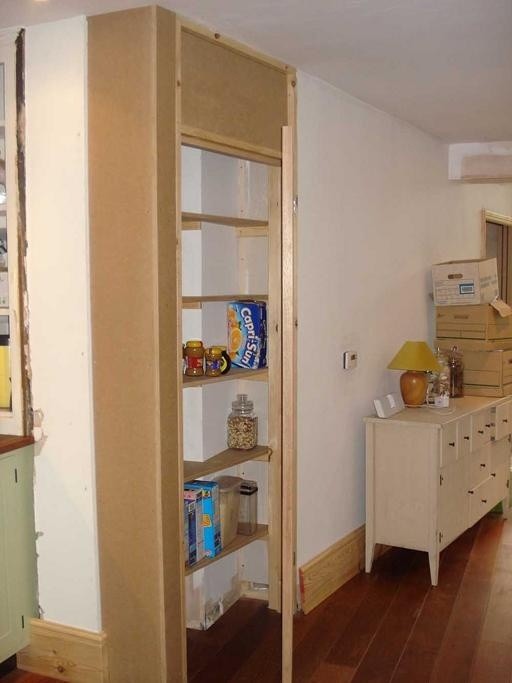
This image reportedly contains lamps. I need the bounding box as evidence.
[386,339,445,409]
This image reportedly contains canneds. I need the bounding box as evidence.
[185,341,231,378]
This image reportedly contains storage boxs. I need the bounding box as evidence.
[428,257,512,397]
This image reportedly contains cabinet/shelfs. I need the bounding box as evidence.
[0,5,298,683]
[365,391,512,587]
